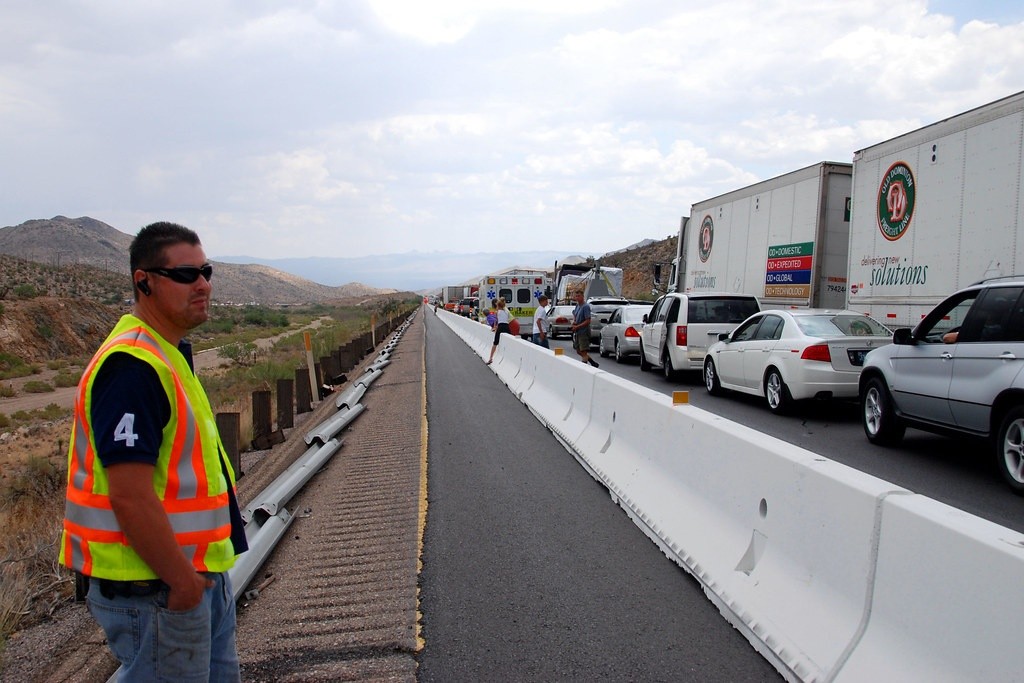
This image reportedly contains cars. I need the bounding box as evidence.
[598,304,654,364]
[427,285,479,320]
[701,307,898,417]
[544,305,578,340]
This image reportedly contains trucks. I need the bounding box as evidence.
[649,159,855,325]
[552,264,624,307]
[442,285,471,307]
[843,91,1023,345]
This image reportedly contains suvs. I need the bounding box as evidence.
[571,296,631,350]
[857,272,1024,500]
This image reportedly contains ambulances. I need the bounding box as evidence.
[476,272,548,341]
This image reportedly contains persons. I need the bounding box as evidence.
[433,302,439,316]
[943,296,1008,344]
[481,297,520,366]
[572,290,600,368]
[54,224,250,683]
[533,295,549,349]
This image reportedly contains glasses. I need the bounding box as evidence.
[142,264,212,285]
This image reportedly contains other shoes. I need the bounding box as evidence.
[486,360,492,365]
[593,363,599,368]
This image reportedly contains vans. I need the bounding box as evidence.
[638,290,763,384]
